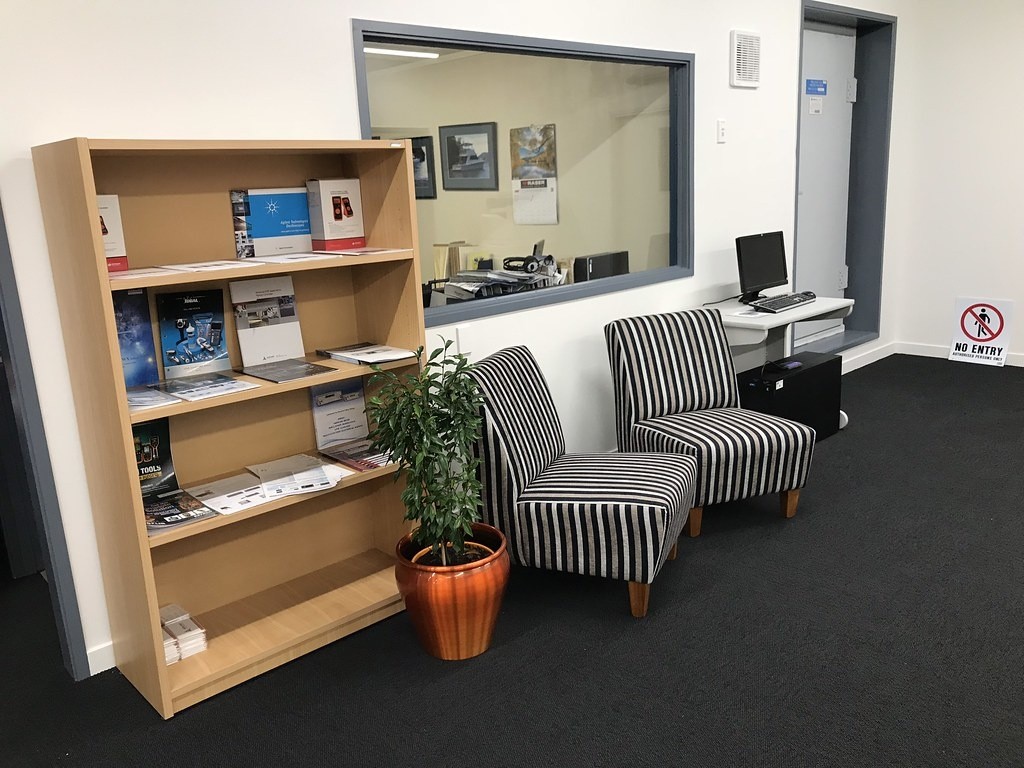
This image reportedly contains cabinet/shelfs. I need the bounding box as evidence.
[31,138,431,722]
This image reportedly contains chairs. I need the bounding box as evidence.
[452,344,701,619]
[533,239,545,257]
[604,307,817,540]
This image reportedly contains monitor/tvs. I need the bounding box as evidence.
[533,241,546,256]
[736,231,788,304]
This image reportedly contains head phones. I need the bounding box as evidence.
[503,256,539,273]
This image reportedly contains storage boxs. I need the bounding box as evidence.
[96,195,130,273]
[305,177,365,251]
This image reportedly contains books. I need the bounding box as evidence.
[450,261,571,300]
[309,374,406,472]
[228,247,423,384]
[131,416,219,537]
[112,286,261,412]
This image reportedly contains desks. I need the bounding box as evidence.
[722,293,857,437]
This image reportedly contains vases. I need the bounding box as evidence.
[395,523,512,662]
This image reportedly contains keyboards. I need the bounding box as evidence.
[749,292,816,313]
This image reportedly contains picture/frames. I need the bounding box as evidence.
[394,136,437,200]
[439,122,498,191]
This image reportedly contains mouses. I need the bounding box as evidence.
[802,290,816,299]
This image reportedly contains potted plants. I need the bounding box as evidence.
[356,333,494,570]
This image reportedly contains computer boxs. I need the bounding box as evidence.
[739,351,843,444]
[574,251,629,284]
